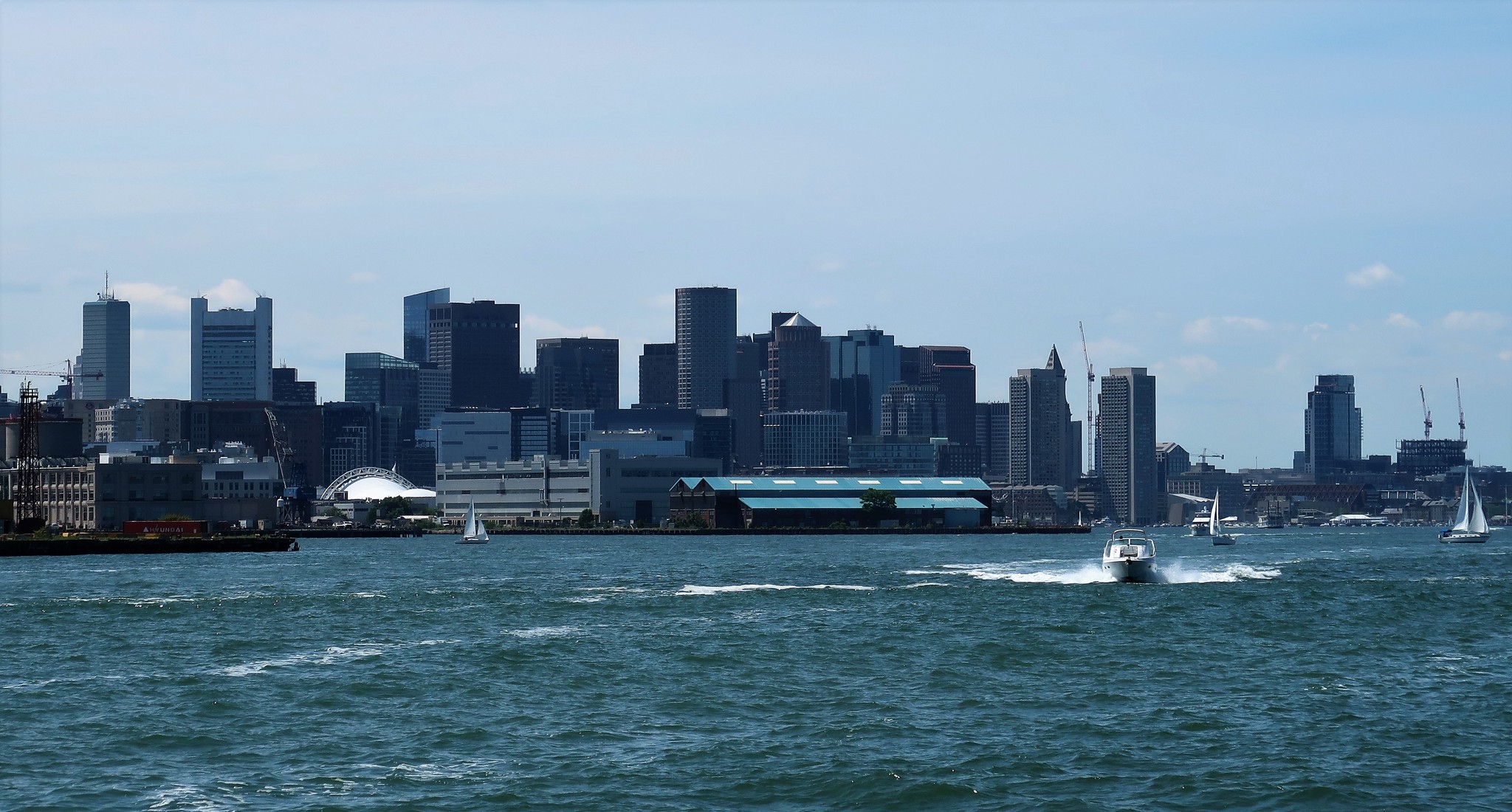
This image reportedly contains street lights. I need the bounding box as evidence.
[557,498,564,521]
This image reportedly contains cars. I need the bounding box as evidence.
[375,523,388,529]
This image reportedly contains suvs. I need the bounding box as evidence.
[332,521,353,529]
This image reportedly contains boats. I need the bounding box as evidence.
[1360,524,1367,528]
[1284,522,1293,528]
[1153,520,1189,527]
[1102,529,1158,583]
[1223,520,1258,529]
[1257,501,1284,529]
[1372,523,1377,527]
[1320,523,1347,528]
[1190,506,1218,536]
[1090,516,1118,529]
[1297,524,1303,528]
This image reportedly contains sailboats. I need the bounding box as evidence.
[1209,485,1237,546]
[1438,464,1492,544]
[454,498,490,544]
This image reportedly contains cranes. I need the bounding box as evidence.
[1078,320,1096,478]
[1189,447,1224,463]
[1455,377,1466,442]
[0,358,105,400]
[1420,384,1433,441]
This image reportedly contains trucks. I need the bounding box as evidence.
[438,517,450,527]
